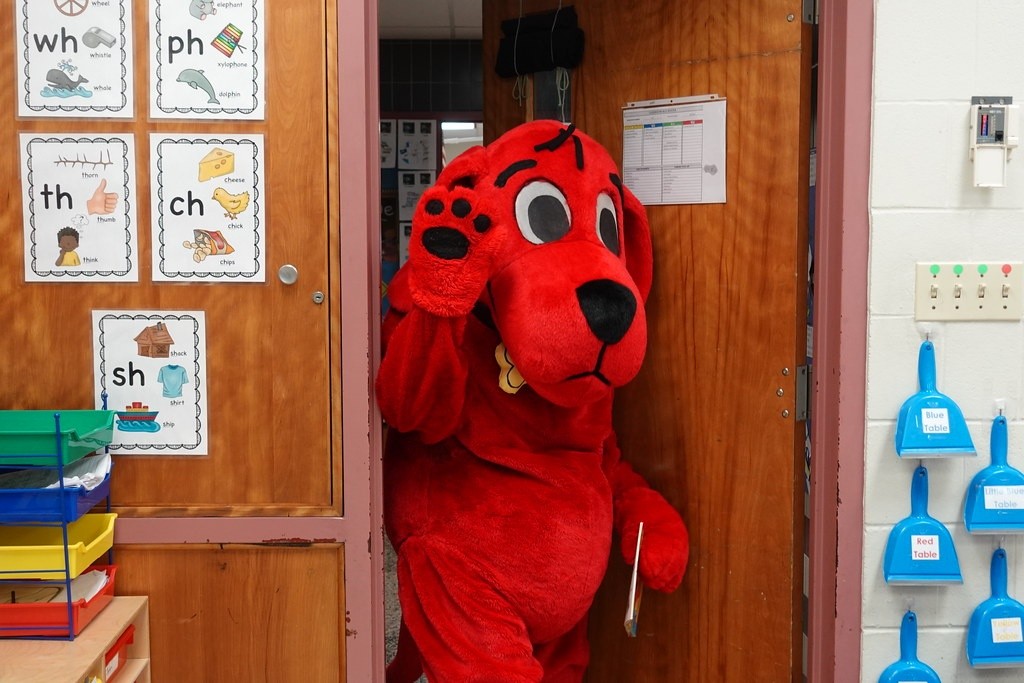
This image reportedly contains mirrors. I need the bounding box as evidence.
[438,120,484,174]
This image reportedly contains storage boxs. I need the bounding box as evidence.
[0,409,117,637]
[105,622,135,683]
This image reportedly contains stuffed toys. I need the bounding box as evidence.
[374,119,690,683]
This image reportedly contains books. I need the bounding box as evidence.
[624,522,643,638]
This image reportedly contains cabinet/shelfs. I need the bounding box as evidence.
[0,594,152,682]
[0,407,120,639]
